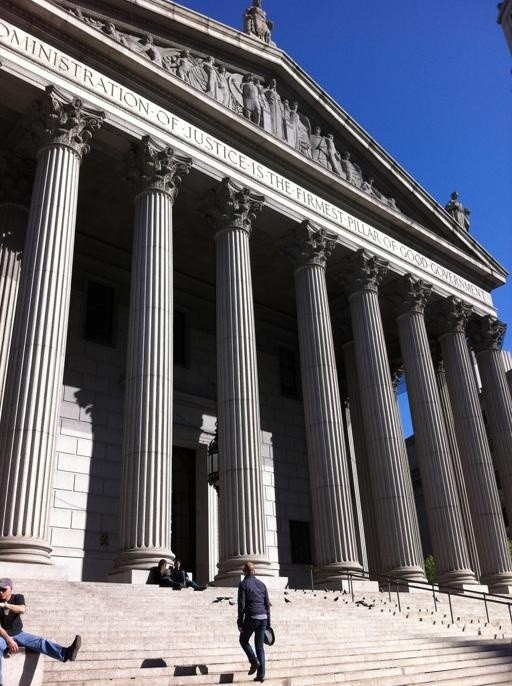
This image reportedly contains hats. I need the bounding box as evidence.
[0,578,13,588]
[264,626,275,646]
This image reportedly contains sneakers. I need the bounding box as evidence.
[254,677,264,681]
[67,635,81,661]
[195,583,209,590]
[248,661,260,675]
[174,584,182,590]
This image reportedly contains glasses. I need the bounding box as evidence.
[1,588,11,593]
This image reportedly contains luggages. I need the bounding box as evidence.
[172,571,187,587]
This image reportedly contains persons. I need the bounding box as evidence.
[238,561,271,682]
[0,578,81,686]
[146,559,208,591]
